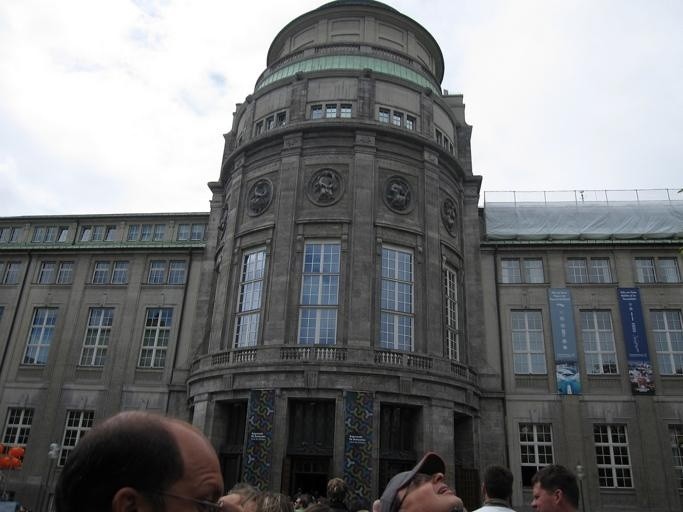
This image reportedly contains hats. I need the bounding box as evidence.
[380,452,446,512]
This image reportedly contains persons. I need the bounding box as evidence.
[54,410,224,512]
[390,178,407,208]
[530,464,579,512]
[251,181,269,210]
[446,202,455,230]
[380,453,464,512]
[219,478,382,512]
[472,467,517,512]
[317,171,335,202]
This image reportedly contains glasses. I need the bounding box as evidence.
[396,473,432,509]
[140,488,223,511]
